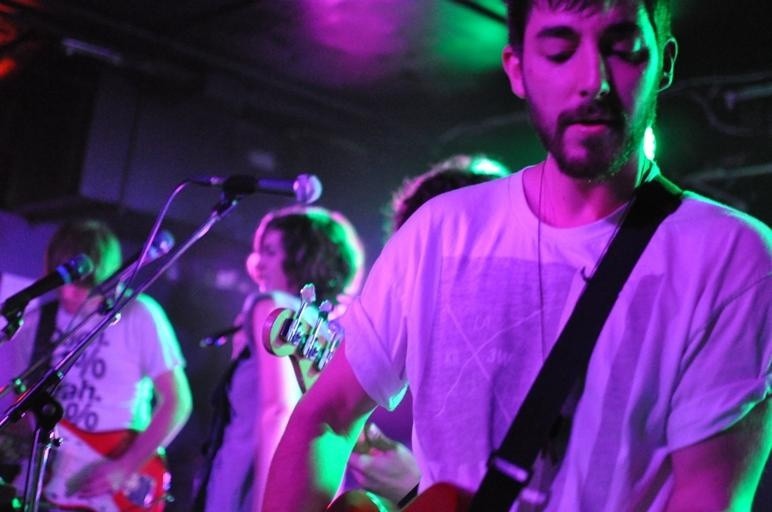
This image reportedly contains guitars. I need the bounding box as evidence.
[3,419,170,512]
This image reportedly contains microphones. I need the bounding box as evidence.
[98,230,175,295]
[5,253,94,312]
[188,173,323,204]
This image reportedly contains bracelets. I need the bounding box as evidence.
[394,483,425,508]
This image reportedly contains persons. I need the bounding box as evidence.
[259,0,772,512]
[346,153,512,512]
[202,204,366,512]
[11,213,194,512]
